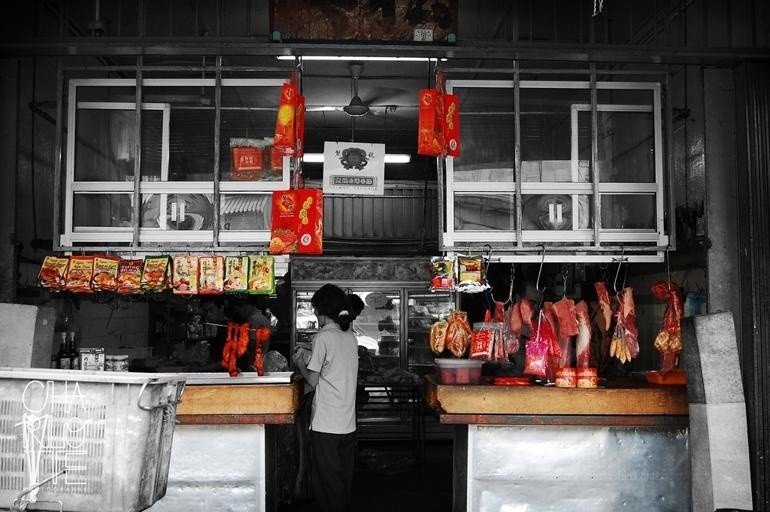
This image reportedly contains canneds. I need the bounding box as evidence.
[104,354,129,371]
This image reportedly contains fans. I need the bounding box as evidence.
[342,64,416,125]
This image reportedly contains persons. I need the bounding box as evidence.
[232,293,291,372]
[345,293,364,321]
[180,297,251,373]
[291,280,362,510]
[486,280,542,377]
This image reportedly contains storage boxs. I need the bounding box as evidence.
[435,359,484,385]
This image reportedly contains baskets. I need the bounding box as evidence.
[0,366,187,512]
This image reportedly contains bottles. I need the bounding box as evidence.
[105,355,129,373]
[69,332,79,369]
[58,331,71,369]
[52,352,57,369]
